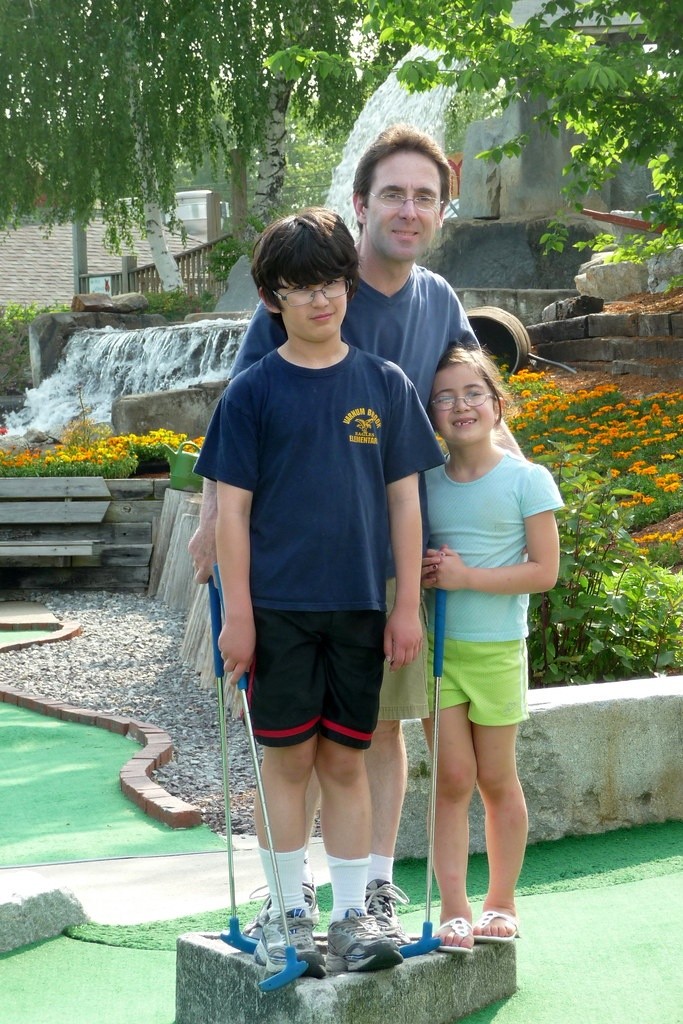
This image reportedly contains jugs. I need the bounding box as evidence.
[163,442,203,493]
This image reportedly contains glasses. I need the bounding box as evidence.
[367,189,445,212]
[272,278,353,307]
[429,390,493,411]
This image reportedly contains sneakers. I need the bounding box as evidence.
[325,914,404,973]
[365,879,412,946]
[243,875,320,938]
[254,907,326,975]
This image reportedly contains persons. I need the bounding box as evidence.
[185,127,524,951]
[193,209,446,977]
[420,346,566,954]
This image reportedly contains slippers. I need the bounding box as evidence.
[474,910,520,943]
[434,917,474,954]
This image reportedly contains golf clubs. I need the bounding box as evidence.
[208,573,261,954]
[398,586,449,958]
[215,566,309,991]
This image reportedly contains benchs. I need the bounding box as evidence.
[0,477,112,558]
